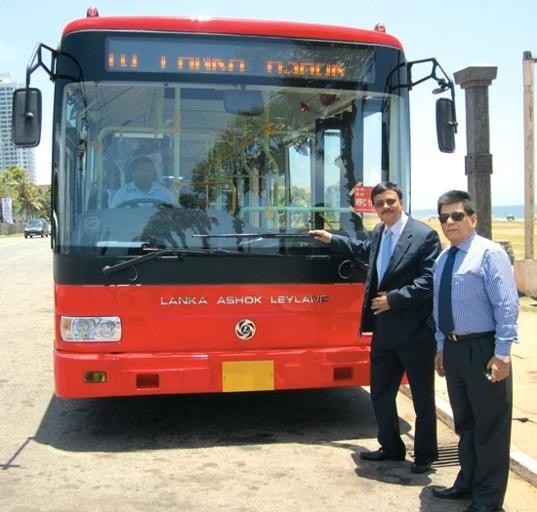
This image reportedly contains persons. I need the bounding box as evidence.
[109,158,177,208]
[430,189,519,510]
[308,182,439,474]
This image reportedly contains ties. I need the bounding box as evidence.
[380,229,393,281]
[438,247,459,336]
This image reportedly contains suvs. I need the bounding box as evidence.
[23,220,49,239]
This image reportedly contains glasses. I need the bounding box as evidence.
[440,212,465,223]
[376,199,397,207]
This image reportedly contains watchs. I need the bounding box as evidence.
[494,353,512,364]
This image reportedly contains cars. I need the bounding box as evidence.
[10,8,454,398]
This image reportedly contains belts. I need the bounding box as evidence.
[448,331,496,342]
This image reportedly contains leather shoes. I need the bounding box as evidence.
[433,487,473,499]
[465,503,497,512]
[360,447,406,460]
[412,461,433,473]
